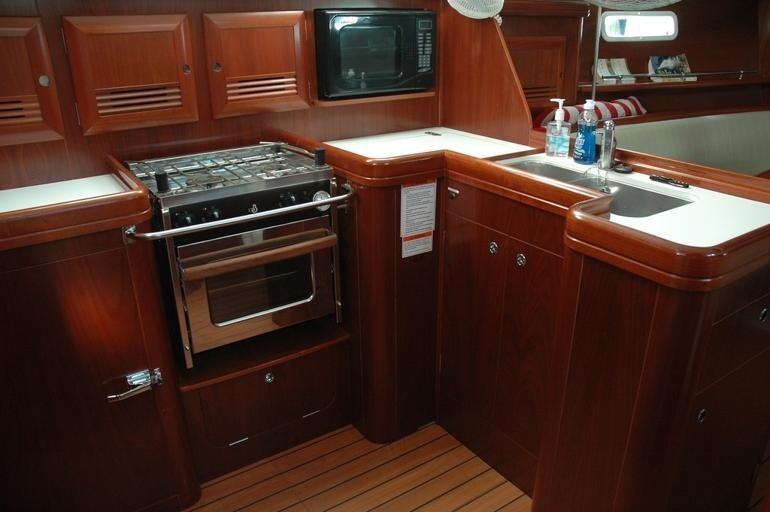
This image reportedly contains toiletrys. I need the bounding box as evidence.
[545,97,571,156]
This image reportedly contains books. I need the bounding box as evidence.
[591,58,636,85]
[648,54,697,83]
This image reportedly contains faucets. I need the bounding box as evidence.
[590,121,616,170]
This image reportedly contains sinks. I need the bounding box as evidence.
[502,159,601,185]
[565,175,695,219]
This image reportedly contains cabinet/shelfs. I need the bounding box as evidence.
[1,15,65,150]
[432,178,568,498]
[1,212,202,510]
[58,10,317,137]
[178,327,356,485]
[531,246,770,512]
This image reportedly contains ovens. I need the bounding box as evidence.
[175,213,338,355]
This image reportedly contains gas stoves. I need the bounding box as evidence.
[126,139,334,247]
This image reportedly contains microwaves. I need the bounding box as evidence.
[313,8,437,102]
